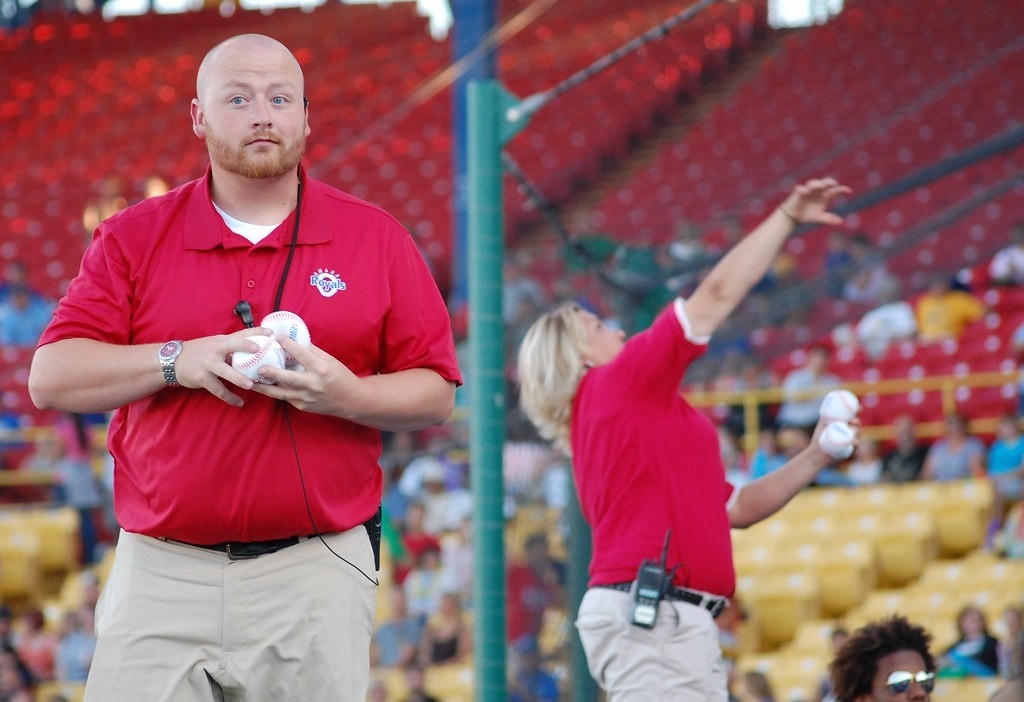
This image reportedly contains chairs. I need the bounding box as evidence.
[0,482,1024,702]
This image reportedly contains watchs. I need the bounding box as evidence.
[158,340,185,386]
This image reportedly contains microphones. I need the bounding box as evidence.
[236,301,253,326]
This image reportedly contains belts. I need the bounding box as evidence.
[601,579,724,618]
[177,534,323,560]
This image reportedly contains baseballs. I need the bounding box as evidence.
[231,310,313,384]
[820,388,862,461]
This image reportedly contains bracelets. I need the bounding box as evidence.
[778,204,802,227]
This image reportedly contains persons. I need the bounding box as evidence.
[517,176,862,702]
[832,614,939,702]
[28,33,464,702]
[0,176,1023,701]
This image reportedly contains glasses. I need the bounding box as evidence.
[884,668,936,695]
